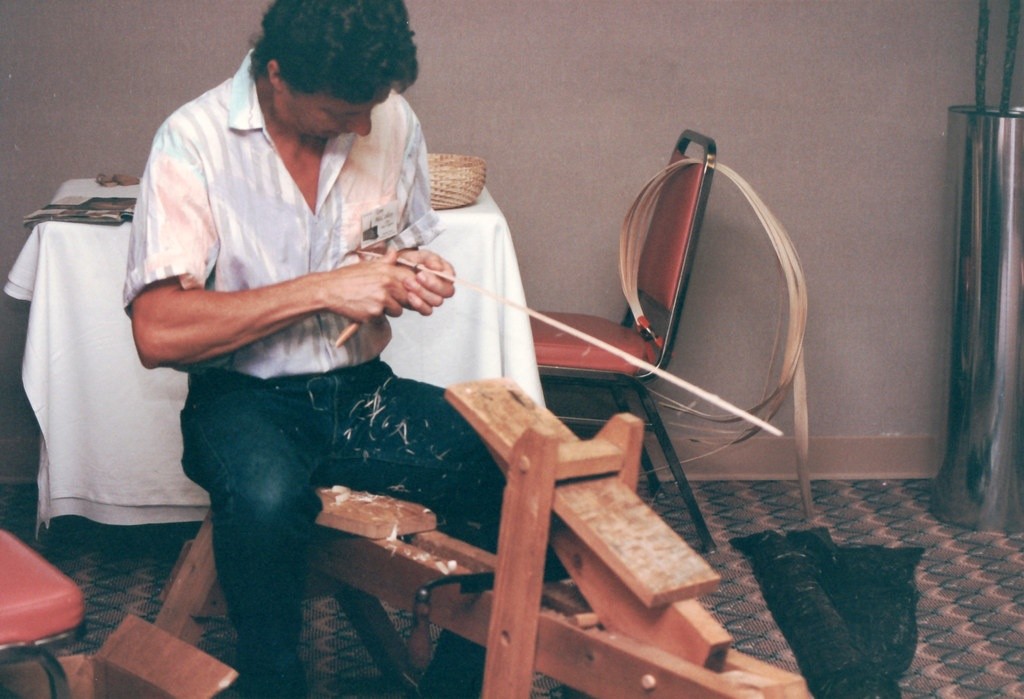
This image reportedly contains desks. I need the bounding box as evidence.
[2,177,546,539]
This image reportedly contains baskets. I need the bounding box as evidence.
[426,153,487,210]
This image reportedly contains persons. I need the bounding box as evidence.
[123,0,508,699]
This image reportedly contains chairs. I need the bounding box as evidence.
[528,131,720,559]
[0,530,87,699]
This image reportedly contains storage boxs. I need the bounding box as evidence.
[1,610,240,699]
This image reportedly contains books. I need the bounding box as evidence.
[22,195,137,231]
